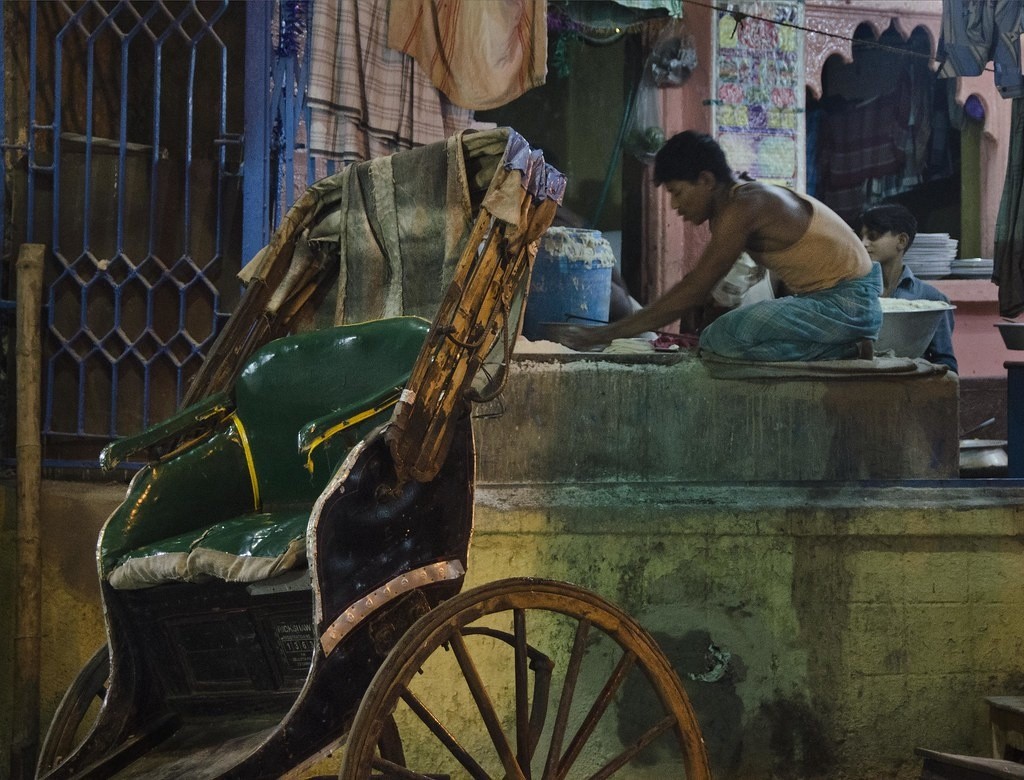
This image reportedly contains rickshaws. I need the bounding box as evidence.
[36,126,714,780]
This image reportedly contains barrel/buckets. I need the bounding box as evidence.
[958,440,1007,469]
[521,226,614,342]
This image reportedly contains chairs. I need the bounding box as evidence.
[94,318,437,593]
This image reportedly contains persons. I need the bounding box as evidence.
[861,205,958,374]
[561,130,883,361]
[680,170,781,336]
[550,207,658,343]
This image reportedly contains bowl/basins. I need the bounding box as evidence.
[993,322,1024,351]
[537,318,613,352]
[871,289,958,358]
